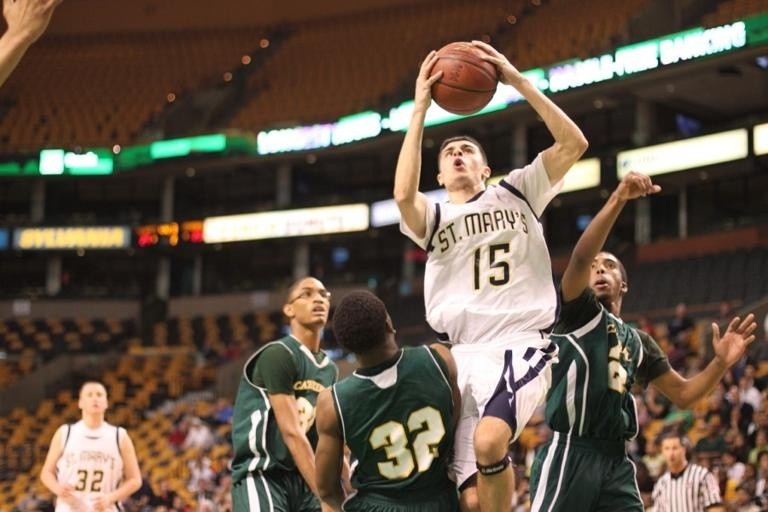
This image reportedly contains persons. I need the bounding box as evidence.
[38,377,145,512]
[0,0,63,90]
[227,273,359,511]
[682,363,765,512]
[13,397,233,512]
[646,433,722,511]
[310,285,466,512]
[503,444,532,511]
[629,301,749,383]
[389,37,593,512]
[623,374,693,511]
[526,170,760,512]
[522,402,553,480]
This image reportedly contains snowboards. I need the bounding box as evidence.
[428,42,499,114]
